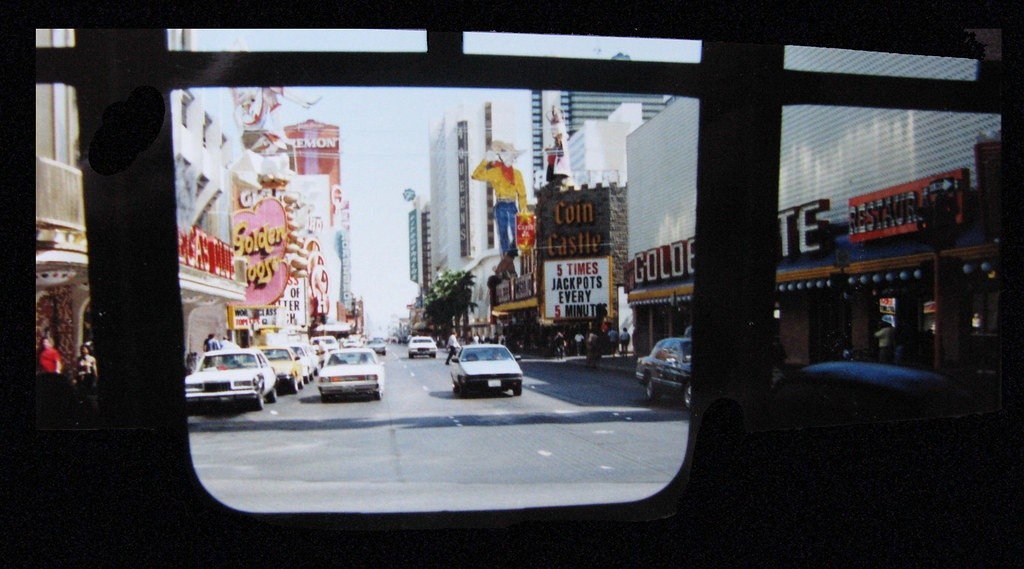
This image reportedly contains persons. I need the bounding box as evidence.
[38,336,98,391]
[444,325,630,365]
[185,333,231,375]
[874,321,893,365]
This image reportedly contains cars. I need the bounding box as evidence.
[343,339,386,355]
[270,343,320,384]
[450,344,523,398]
[408,336,437,359]
[318,348,386,403]
[242,346,304,395]
[185,348,277,415]
[310,336,338,363]
[635,337,692,411]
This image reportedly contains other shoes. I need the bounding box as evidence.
[446,362,449,364]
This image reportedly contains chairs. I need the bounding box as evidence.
[467,354,478,361]
